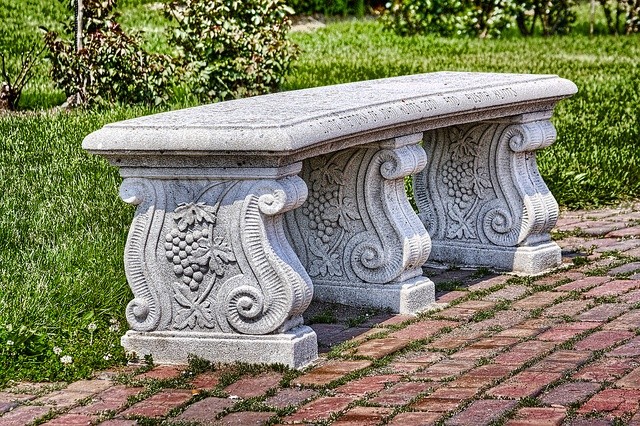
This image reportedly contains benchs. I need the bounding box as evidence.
[81,71,593,369]
